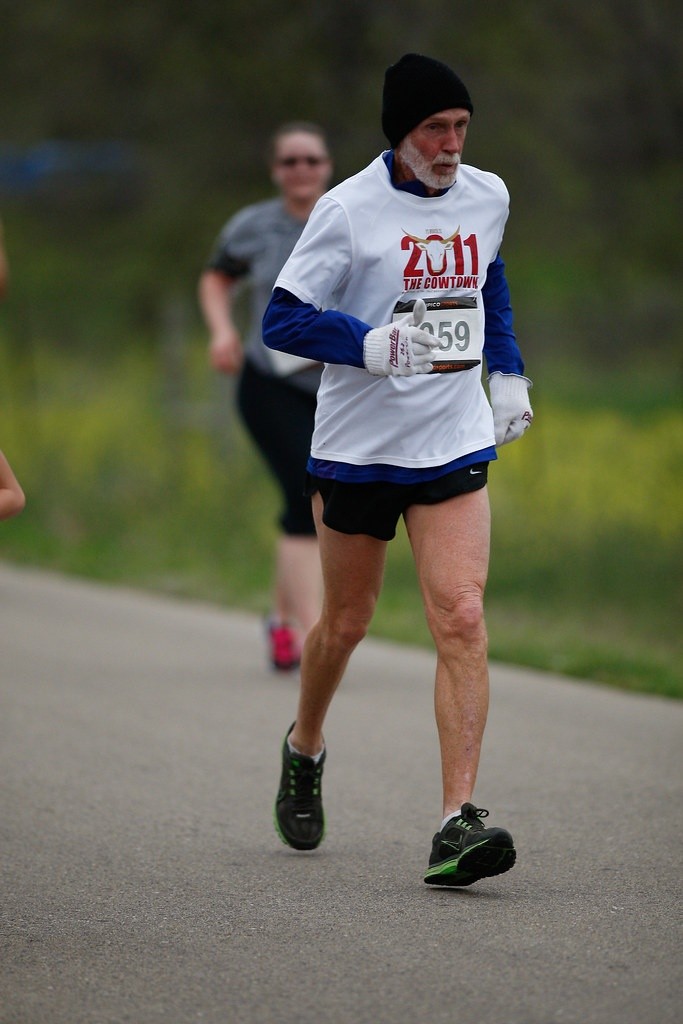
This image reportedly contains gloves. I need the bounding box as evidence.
[490,373,533,448]
[362,298,440,377]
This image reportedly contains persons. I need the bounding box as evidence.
[262,52,534,886]
[199,121,331,675]
[0,449,26,522]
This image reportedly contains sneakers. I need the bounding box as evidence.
[273,721,326,850]
[424,802,516,886]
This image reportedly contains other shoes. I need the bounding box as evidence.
[268,617,301,669]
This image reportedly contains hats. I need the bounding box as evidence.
[382,54,473,149]
